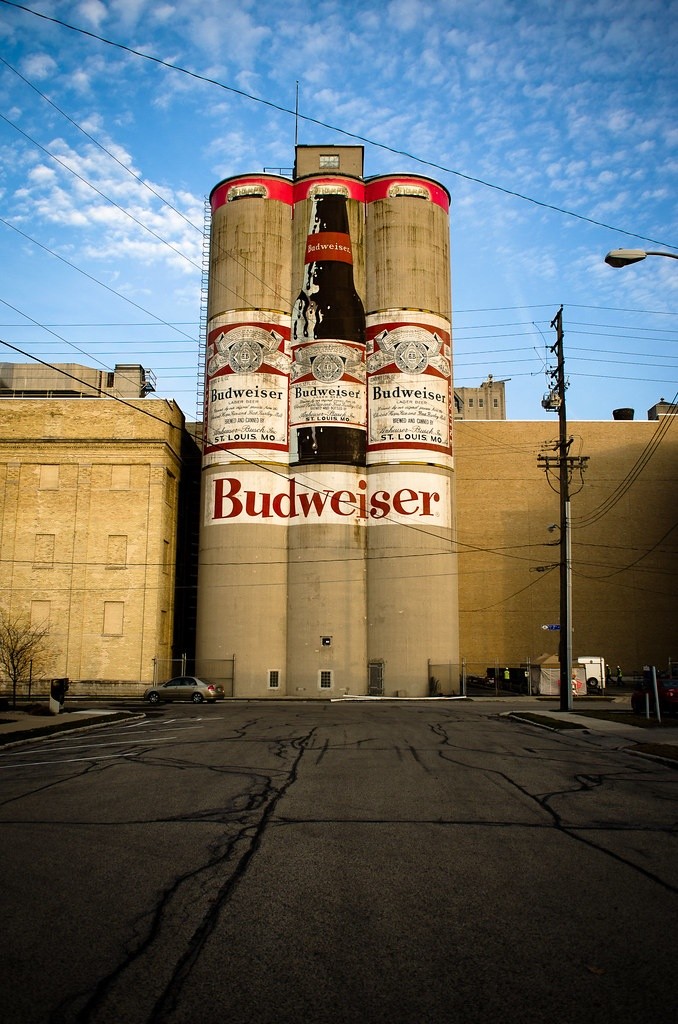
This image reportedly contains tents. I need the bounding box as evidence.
[529,652,587,696]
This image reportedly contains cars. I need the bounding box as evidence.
[143,676,225,704]
[631,678,678,716]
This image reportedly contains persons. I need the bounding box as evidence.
[615,666,626,686]
[503,667,512,690]
[605,664,616,683]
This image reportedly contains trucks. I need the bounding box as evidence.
[578,656,606,695]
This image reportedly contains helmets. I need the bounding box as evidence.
[506,667,508,669]
[606,664,609,666]
[616,666,620,669]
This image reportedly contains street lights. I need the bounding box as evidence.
[547,523,574,711]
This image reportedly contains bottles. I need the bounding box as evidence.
[504,667,510,679]
[288,184,370,467]
[225,182,268,203]
[386,182,431,202]
